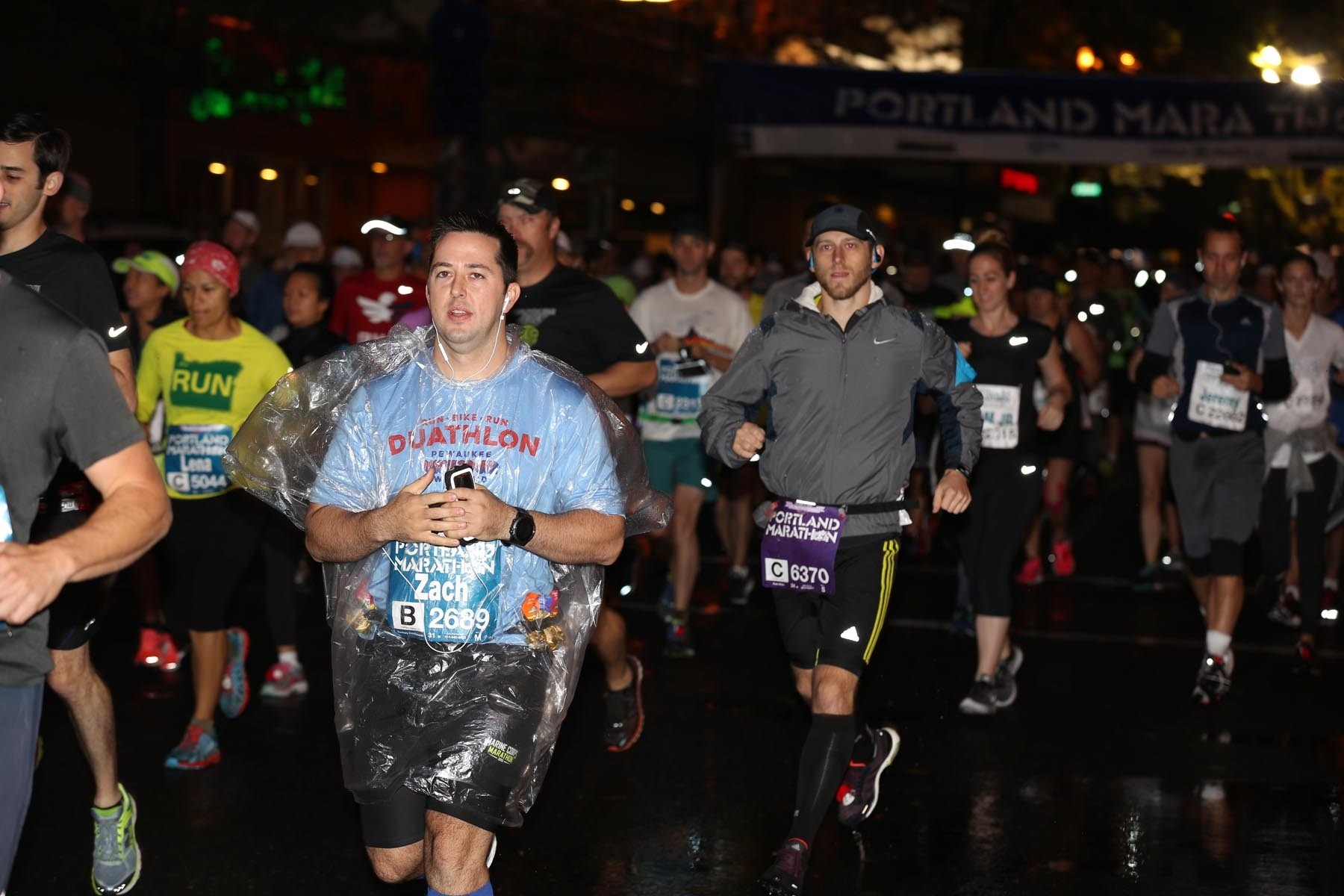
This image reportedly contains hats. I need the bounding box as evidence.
[496,179,561,216]
[284,224,321,248]
[113,250,179,292]
[805,204,871,247]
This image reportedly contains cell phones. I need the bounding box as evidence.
[673,360,708,378]
[444,464,479,546]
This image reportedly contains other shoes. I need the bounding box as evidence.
[1192,647,1235,703]
[662,612,695,657]
[1131,568,1182,591]
[993,645,1023,707]
[656,573,676,622]
[1016,558,1041,587]
[1052,539,1074,576]
[961,673,998,717]
[726,568,755,606]
[1269,590,1305,624]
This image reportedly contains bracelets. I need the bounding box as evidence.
[955,467,971,480]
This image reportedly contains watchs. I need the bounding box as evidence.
[499,506,535,547]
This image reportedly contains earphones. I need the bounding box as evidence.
[502,297,510,313]
[807,250,814,269]
[874,245,882,264]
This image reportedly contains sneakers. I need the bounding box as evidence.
[602,656,645,754]
[219,628,250,719]
[90,780,142,895]
[839,726,900,823]
[167,719,220,767]
[261,663,310,697]
[758,837,808,896]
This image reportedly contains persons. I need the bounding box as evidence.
[0,110,1344,896]
